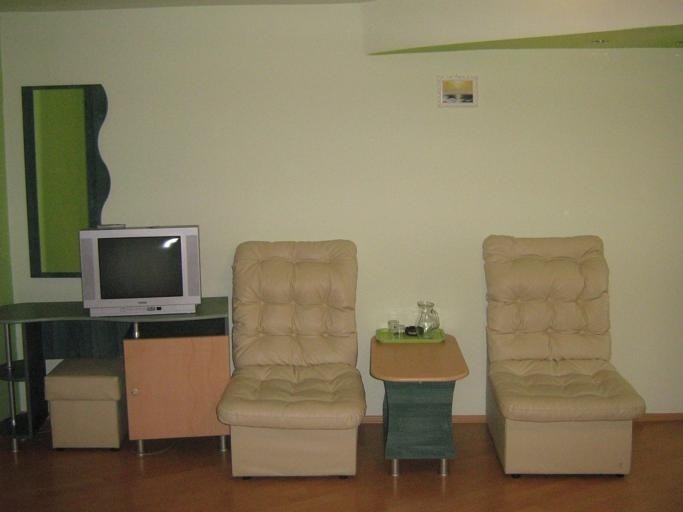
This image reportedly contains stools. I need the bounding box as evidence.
[42,357,124,454]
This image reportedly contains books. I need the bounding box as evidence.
[96,222,126,229]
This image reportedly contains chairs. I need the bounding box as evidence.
[215,240,365,481]
[483,234,647,480]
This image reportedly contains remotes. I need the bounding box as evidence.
[96,223,125,229]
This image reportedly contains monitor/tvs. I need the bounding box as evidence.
[79,225,202,317]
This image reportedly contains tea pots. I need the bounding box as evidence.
[415,300,441,340]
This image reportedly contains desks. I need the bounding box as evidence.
[1,296,232,464]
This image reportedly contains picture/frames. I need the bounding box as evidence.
[437,73,480,109]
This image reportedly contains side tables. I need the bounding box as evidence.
[369,332,468,479]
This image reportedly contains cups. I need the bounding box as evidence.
[393,325,405,340]
[387,319,400,334]
